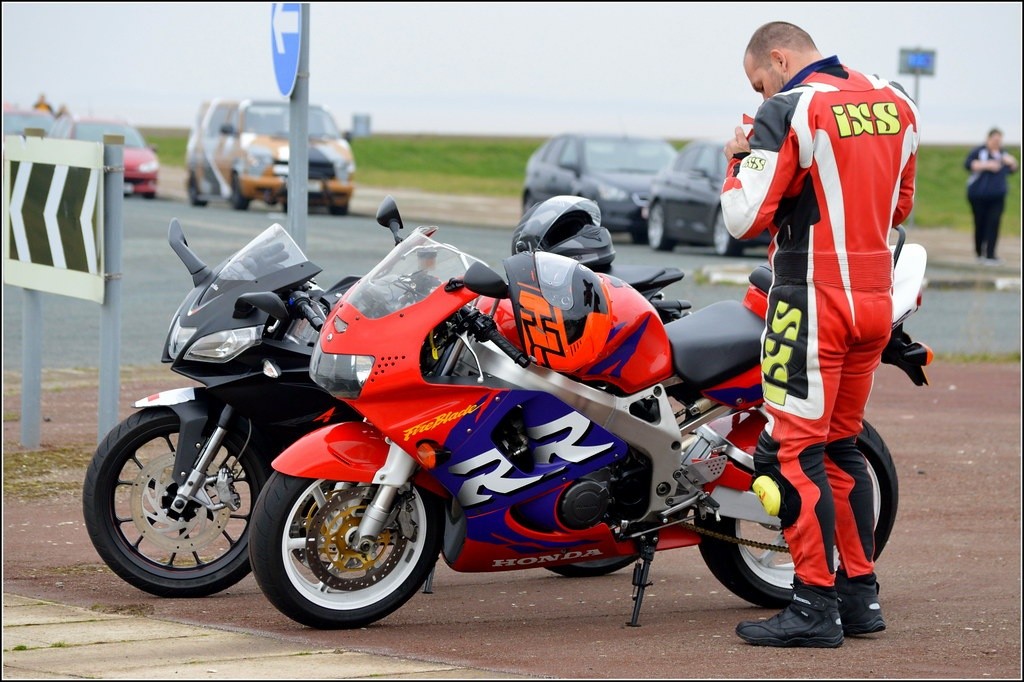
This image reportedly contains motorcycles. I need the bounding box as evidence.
[83,210,693,600]
[247,197,933,631]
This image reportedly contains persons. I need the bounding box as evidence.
[964,127,1020,266]
[722,21,921,647]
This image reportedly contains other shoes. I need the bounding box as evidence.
[975,255,1006,269]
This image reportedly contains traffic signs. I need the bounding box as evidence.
[270,2,303,96]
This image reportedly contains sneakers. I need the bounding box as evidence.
[836,567,887,635]
[735,575,844,649]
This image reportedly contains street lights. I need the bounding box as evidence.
[896,47,936,111]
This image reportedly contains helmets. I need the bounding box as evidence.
[510,194,616,269]
[503,250,613,374]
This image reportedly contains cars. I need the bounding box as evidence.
[519,134,680,246]
[646,137,771,256]
[2,108,163,199]
[185,99,355,214]
